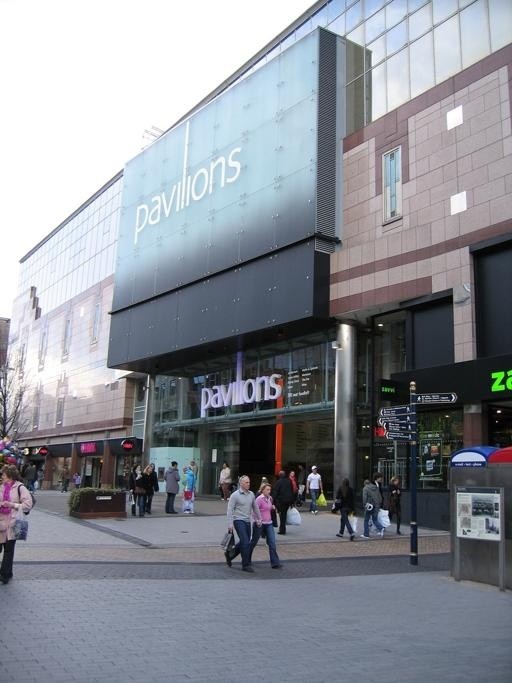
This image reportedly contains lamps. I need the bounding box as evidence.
[142,384,150,391]
[331,332,339,350]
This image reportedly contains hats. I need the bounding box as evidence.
[312,466,317,469]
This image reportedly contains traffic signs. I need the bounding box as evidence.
[380,404,416,415]
[384,431,416,441]
[376,415,416,426]
[410,393,457,405]
[384,422,417,432]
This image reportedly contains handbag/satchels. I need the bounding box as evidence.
[21,493,36,513]
[271,509,277,524]
[331,503,340,513]
[8,520,28,540]
[134,487,144,493]
[220,534,234,552]
[184,491,192,500]
[128,494,135,504]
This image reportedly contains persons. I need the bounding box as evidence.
[244,483,283,569]
[0,463,34,584]
[129,457,404,542]
[224,474,263,572]
[16,461,82,494]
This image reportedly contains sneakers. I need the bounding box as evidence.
[336,528,385,541]
[224,552,280,572]
